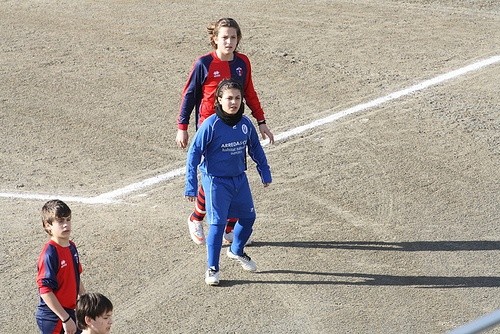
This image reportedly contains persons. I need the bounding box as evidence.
[36,199,87,334]
[184,78,273,285]
[175,18,275,244]
[75,292,113,334]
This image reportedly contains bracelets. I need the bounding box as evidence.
[62,315,71,323]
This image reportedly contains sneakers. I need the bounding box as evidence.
[188,211,205,245]
[223,230,251,245]
[227,246,257,272]
[205,268,219,285]
